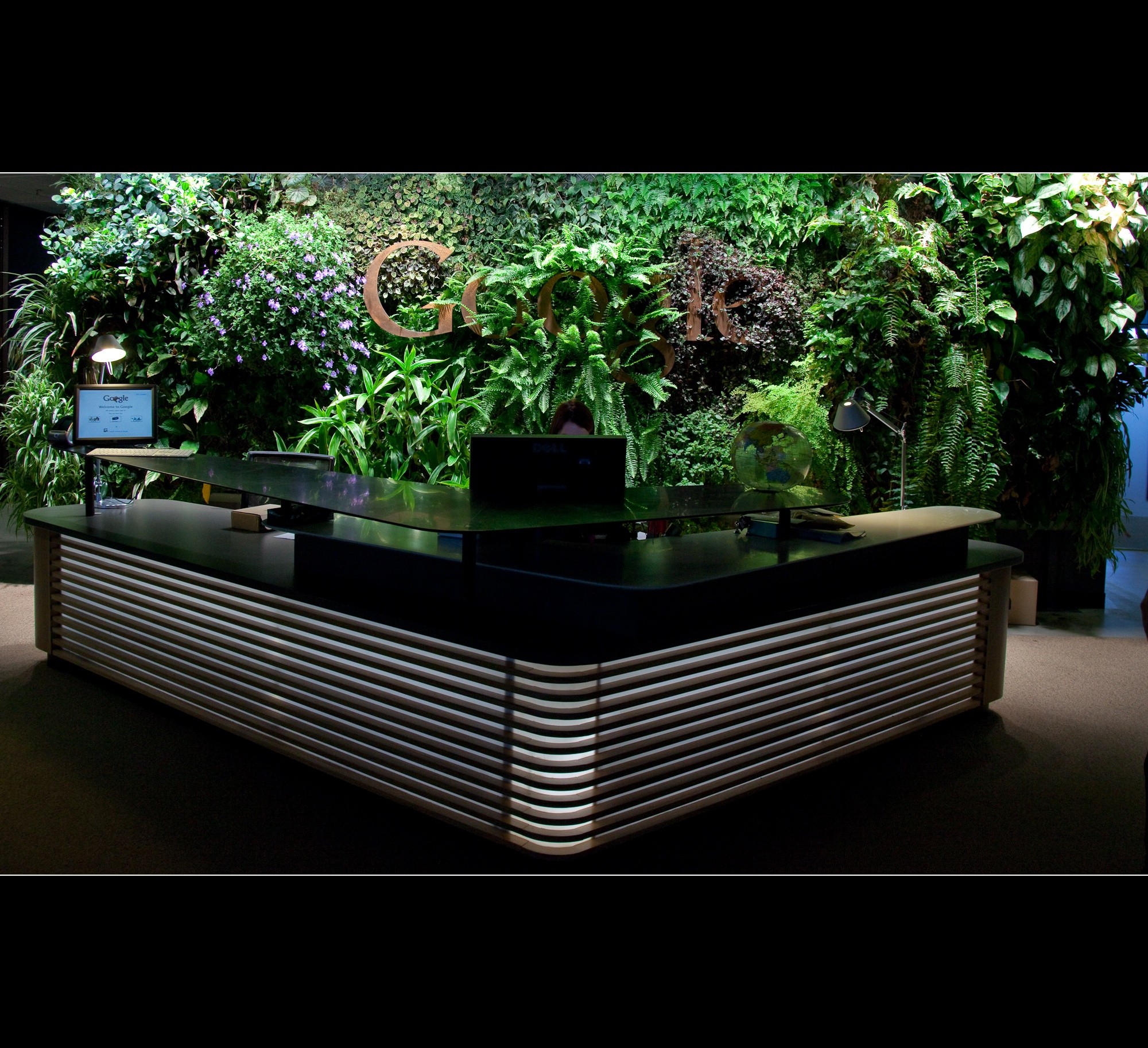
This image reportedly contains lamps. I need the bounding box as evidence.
[91,333,127,385]
[833,399,907,512]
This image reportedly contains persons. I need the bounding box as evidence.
[549,400,595,435]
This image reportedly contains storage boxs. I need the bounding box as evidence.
[1008,575,1038,626]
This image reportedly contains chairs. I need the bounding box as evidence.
[240,450,335,511]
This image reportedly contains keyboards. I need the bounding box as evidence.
[86,448,195,457]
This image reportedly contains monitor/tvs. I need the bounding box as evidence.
[73,384,158,448]
[468,434,628,491]
